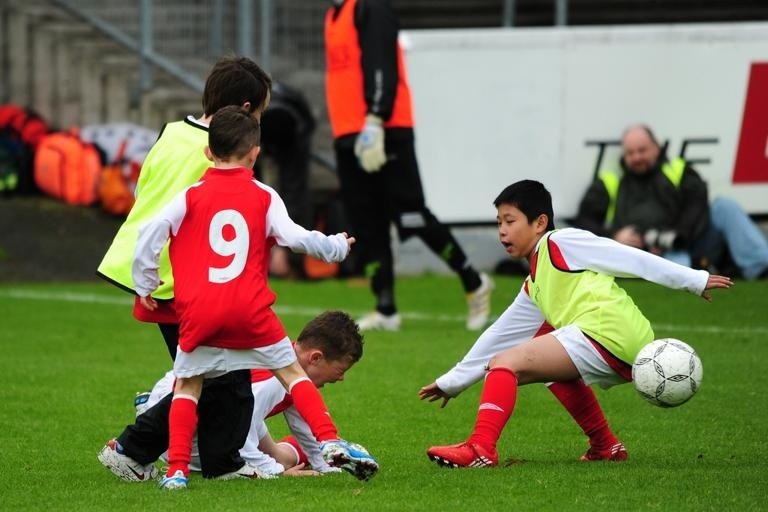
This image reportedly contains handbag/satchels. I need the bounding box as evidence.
[97,158,141,216]
[32,126,103,208]
[0,105,47,146]
[0,125,33,198]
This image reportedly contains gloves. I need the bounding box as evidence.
[354,113,387,172]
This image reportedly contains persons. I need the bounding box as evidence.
[96,56,280,481]
[134,310,364,478]
[569,125,768,282]
[325,0,495,332]
[130,105,379,490]
[419,180,735,468]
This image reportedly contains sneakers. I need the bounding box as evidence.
[318,436,379,482]
[97,437,162,483]
[465,272,495,331]
[355,310,402,332]
[579,441,629,461]
[159,466,190,491]
[217,461,279,481]
[427,440,498,469]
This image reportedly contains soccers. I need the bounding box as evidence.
[630,338,703,407]
